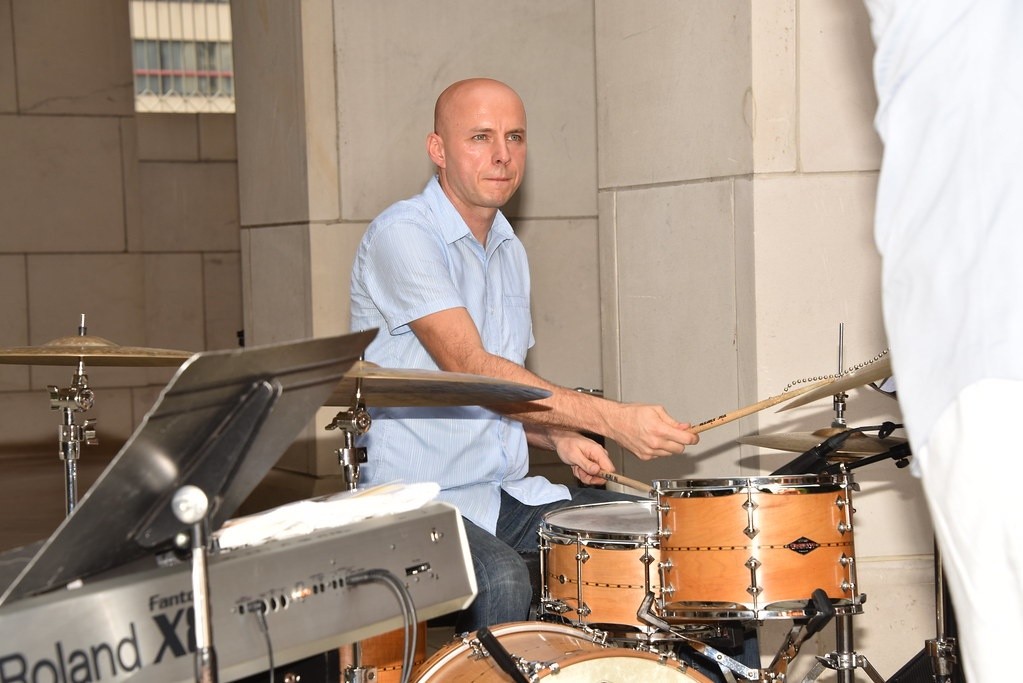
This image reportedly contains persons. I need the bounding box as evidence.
[350,78,699,641]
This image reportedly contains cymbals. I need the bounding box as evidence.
[736,422,911,455]
[0,333,198,367]
[322,359,554,406]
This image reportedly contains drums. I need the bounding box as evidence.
[403,621,714,683]
[654,467,863,622]
[538,497,663,634]
